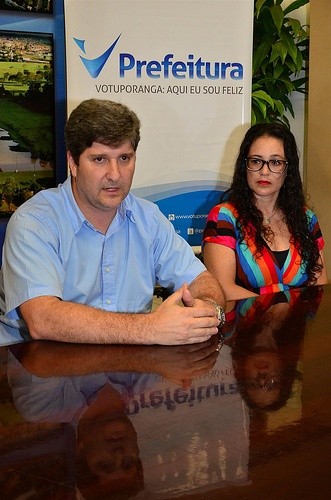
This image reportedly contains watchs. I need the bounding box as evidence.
[200,297,227,330]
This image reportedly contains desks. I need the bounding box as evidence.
[0,284,331,500]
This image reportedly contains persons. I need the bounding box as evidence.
[199,122,329,304]
[214,286,325,412]
[0,98,227,346]
[5,339,222,500]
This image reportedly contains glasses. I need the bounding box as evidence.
[241,376,284,391]
[244,157,289,175]
